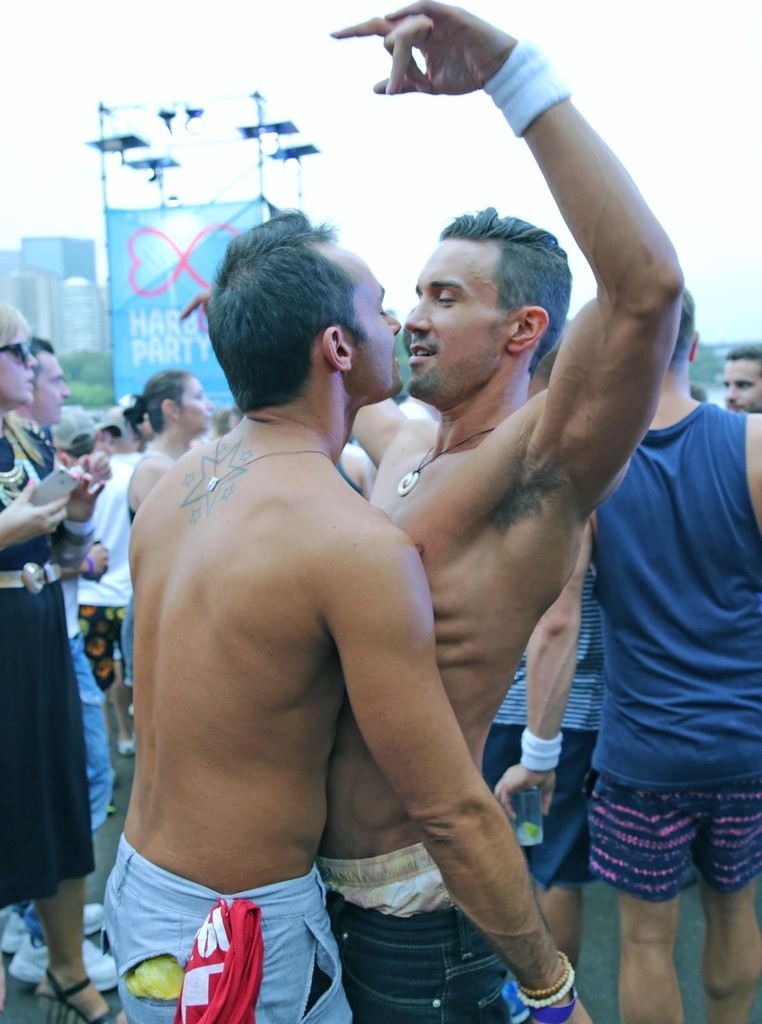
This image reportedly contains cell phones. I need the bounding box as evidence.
[29,469,81,507]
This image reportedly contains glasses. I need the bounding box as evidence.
[0,341,31,362]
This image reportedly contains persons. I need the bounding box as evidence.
[481,350,604,1024]
[0,302,247,1024]
[103,212,593,1024]
[723,343,762,414]
[496,284,762,1024]
[312,0,679,1024]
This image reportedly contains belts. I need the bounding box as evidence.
[0,559,62,593]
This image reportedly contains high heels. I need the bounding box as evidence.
[34,967,125,1024]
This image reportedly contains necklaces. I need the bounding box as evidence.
[205,434,337,492]
[397,426,498,497]
[0,424,43,509]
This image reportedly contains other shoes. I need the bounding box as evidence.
[118,737,135,757]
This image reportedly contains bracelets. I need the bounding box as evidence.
[85,556,93,576]
[517,950,578,1024]
[63,519,96,547]
[519,728,563,771]
[485,41,570,137]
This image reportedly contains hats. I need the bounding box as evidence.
[92,410,122,437]
[48,403,96,451]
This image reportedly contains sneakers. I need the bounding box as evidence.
[7,932,120,993]
[2,902,103,954]
